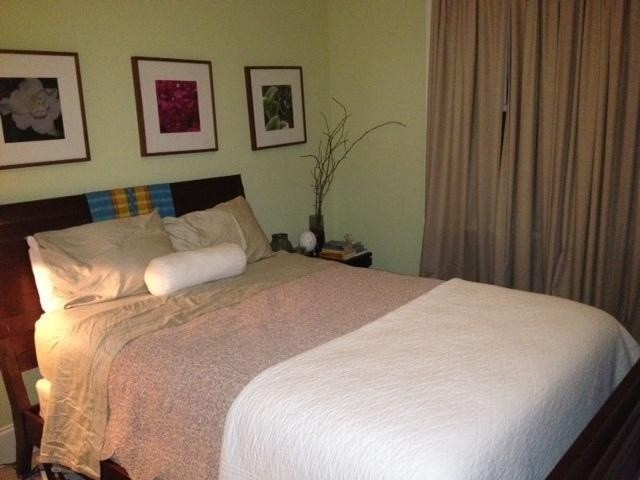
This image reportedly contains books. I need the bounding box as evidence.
[320,251,356,260]
[324,240,352,251]
[322,247,356,255]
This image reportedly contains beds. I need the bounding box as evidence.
[0,172,640,479]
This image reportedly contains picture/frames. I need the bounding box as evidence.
[130,55,218,157]
[0,49,91,170]
[244,66,307,151]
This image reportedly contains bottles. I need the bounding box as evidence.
[269,234,293,253]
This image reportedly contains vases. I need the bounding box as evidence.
[310,214,326,252]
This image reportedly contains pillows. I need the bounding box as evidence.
[26,190,278,310]
